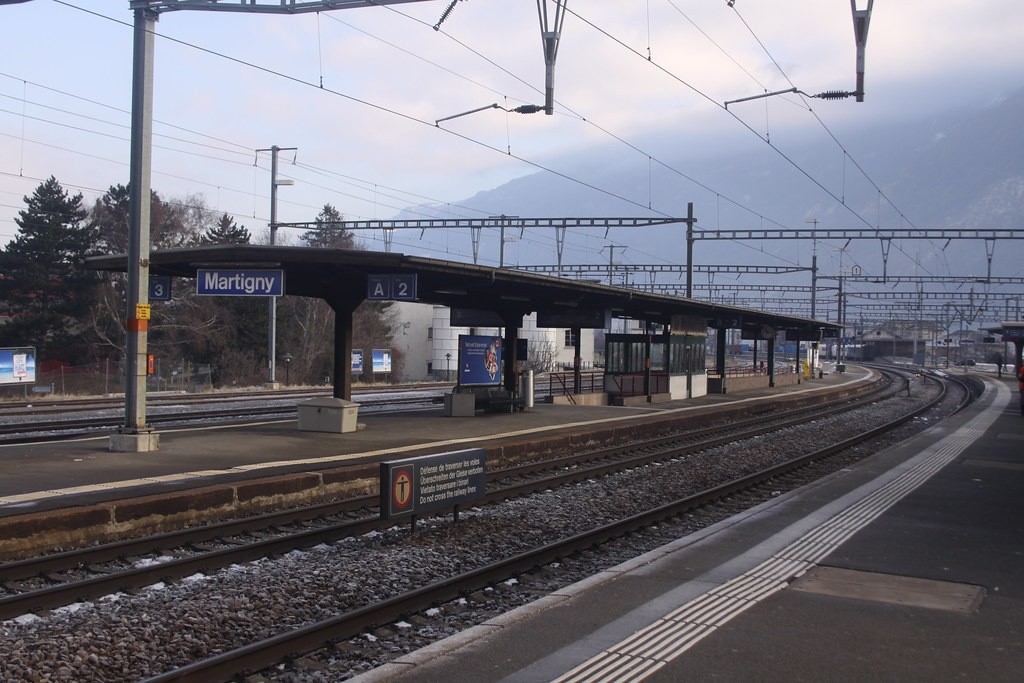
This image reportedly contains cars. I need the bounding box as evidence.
[937,357,951,364]
[957,359,975,366]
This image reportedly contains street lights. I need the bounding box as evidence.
[283,353,293,385]
[445,352,451,382]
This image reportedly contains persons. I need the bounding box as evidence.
[484,339,498,380]
[1016,360,1024,416]
